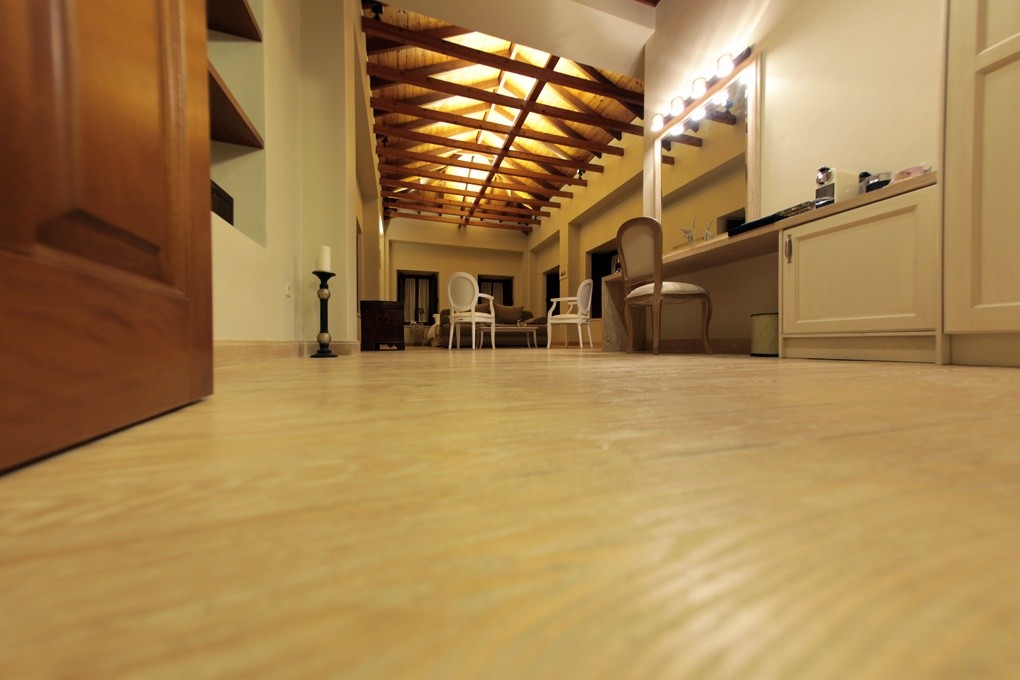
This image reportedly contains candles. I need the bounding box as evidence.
[314,245,331,273]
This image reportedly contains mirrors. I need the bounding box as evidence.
[654,49,761,260]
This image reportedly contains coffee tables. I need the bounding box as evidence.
[476,326,538,349]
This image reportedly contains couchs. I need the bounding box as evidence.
[440,303,546,347]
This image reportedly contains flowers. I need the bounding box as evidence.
[702,220,714,241]
[678,218,696,239]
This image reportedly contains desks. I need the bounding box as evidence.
[601,220,780,353]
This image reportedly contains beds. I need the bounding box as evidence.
[428,324,440,346]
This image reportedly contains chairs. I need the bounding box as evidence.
[547,279,593,348]
[616,216,715,355]
[445,272,496,350]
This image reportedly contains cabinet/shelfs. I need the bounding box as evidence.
[942,0,1020,369]
[778,184,943,363]
[360,299,405,351]
[209,0,267,148]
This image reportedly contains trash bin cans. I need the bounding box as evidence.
[752,313,780,357]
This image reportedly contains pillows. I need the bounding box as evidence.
[433,314,440,324]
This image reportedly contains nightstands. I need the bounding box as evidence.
[410,328,425,346]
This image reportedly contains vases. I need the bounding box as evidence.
[688,240,695,249]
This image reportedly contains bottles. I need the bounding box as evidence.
[517,320,521,327]
